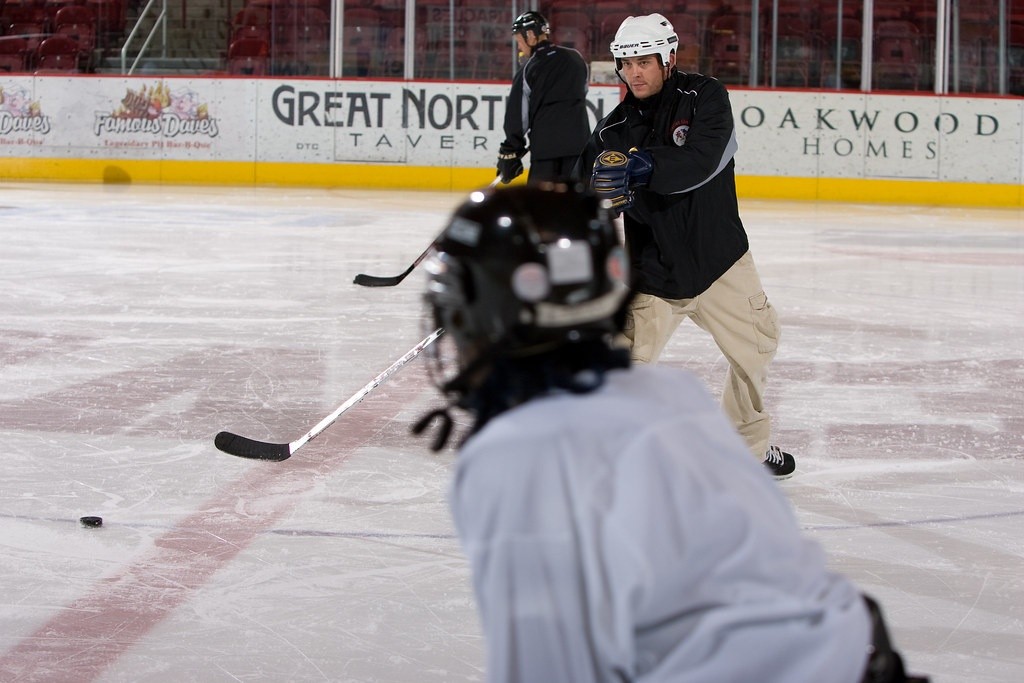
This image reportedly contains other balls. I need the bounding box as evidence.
[78,514,104,530]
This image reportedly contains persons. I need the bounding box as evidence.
[416,182,931,683]
[494,12,590,188]
[570,14,796,480]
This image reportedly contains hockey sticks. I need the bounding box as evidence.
[210,325,447,466]
[351,145,531,289]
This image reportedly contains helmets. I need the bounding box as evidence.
[610,13,679,72]
[511,11,551,35]
[424,187,632,408]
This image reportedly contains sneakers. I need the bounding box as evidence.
[761,445,795,479]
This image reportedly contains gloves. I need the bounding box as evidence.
[496,142,524,186]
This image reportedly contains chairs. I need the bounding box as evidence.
[226,0,1024,94]
[0,0,126,74]
